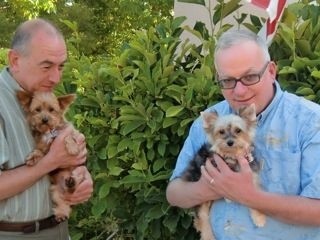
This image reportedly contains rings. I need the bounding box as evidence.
[210,179,214,184]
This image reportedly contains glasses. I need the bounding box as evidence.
[214,62,272,89]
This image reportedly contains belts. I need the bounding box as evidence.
[0,214,59,235]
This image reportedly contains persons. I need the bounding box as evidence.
[166,29,320,240]
[0,19,94,240]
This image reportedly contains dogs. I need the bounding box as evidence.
[15,89,84,222]
[181,102,267,240]
